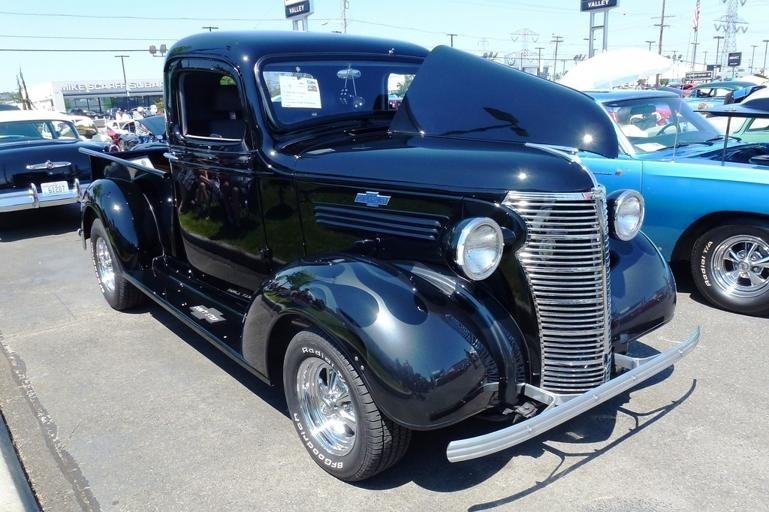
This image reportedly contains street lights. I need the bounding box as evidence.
[114,55,130,90]
[690,42,699,66]
[750,45,758,65]
[448,33,458,49]
[713,36,724,64]
[763,40,769,67]
[534,47,545,76]
[645,40,655,51]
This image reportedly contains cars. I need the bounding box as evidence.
[0,104,166,221]
[77,29,701,484]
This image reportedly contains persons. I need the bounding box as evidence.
[132,106,151,144]
[175,134,243,227]
[114,107,132,120]
[697,89,707,98]
[108,129,122,153]
